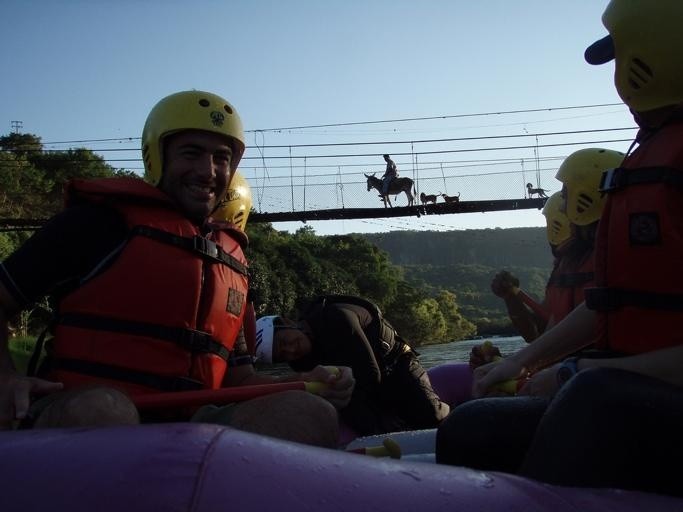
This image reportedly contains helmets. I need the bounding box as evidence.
[542,148,625,247]
[141,89,251,232]
[254,314,283,363]
[602,0,683,112]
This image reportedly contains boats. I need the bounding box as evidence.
[0,358,680,510]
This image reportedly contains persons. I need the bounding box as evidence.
[253,293,452,435]
[375,153,398,203]
[465,146,630,398]
[433,0,682,500]
[0,90,356,451]
[209,173,254,230]
[467,189,584,345]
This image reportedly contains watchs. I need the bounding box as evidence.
[554,357,579,391]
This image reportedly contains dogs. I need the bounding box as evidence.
[526,182,551,198]
[420,193,442,206]
[441,192,461,204]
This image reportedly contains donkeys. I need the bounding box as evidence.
[363,172,416,208]
